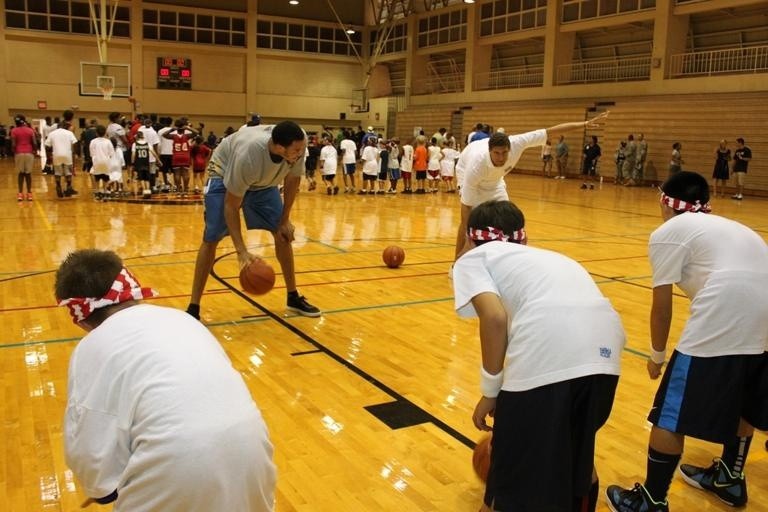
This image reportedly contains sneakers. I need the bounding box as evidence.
[678,458,749,508]
[602,482,671,512]
[55,188,81,200]
[17,193,35,201]
[731,193,744,200]
[580,183,594,190]
[91,178,204,203]
[613,180,624,185]
[285,295,322,317]
[554,175,566,180]
[624,182,635,187]
[308,180,456,195]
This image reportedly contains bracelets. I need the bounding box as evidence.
[650,341,666,364]
[479,366,504,398]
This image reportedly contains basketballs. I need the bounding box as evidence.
[240,262,274,295]
[384,245,404,268]
[473,431,493,481]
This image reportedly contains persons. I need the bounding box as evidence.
[540,134,752,199]
[224,126,236,135]
[452,201,626,512]
[11,107,217,203]
[186,120,321,320]
[252,114,262,120]
[465,123,505,146]
[454,110,612,266]
[53,248,277,512]
[605,170,768,512]
[305,125,462,195]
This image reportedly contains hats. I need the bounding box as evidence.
[368,126,373,130]
[252,115,261,121]
